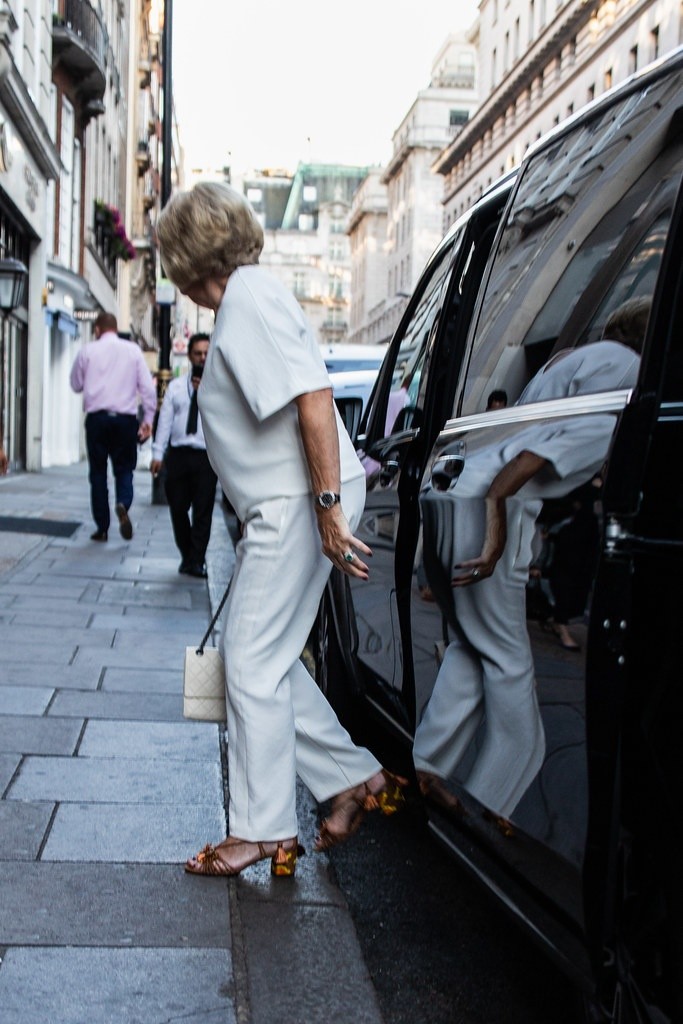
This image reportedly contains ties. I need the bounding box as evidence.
[187,382,198,436]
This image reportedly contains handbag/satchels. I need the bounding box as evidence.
[184,646,227,723]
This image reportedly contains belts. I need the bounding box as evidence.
[94,410,130,421]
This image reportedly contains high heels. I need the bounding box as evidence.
[313,768,407,853]
[184,837,299,877]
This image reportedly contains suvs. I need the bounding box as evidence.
[296,50,682,1024]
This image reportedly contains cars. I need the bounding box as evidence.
[318,340,391,423]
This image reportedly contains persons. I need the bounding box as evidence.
[69,312,159,543]
[151,180,400,883]
[150,332,220,579]
[404,290,663,838]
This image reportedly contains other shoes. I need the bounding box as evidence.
[115,504,133,539]
[557,628,578,650]
[177,561,191,574]
[90,530,107,541]
[188,562,207,577]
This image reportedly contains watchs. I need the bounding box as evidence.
[314,491,341,510]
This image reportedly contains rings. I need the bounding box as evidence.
[344,550,354,562]
[473,568,480,577]
